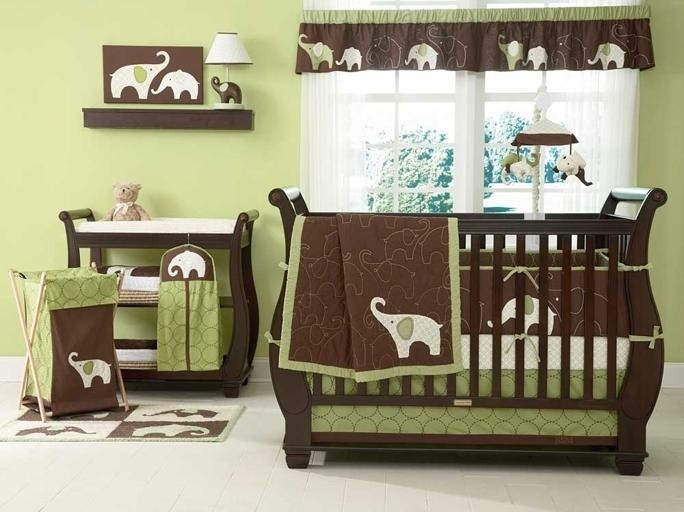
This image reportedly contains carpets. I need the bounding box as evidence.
[0,399,247,443]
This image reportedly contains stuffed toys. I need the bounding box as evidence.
[500,154,539,183]
[100,181,152,222]
[552,150,592,187]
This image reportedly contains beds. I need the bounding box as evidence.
[265,186,669,476]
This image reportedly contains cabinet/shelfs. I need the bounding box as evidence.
[57,208,259,399]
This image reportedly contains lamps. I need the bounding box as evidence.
[203,31,254,109]
